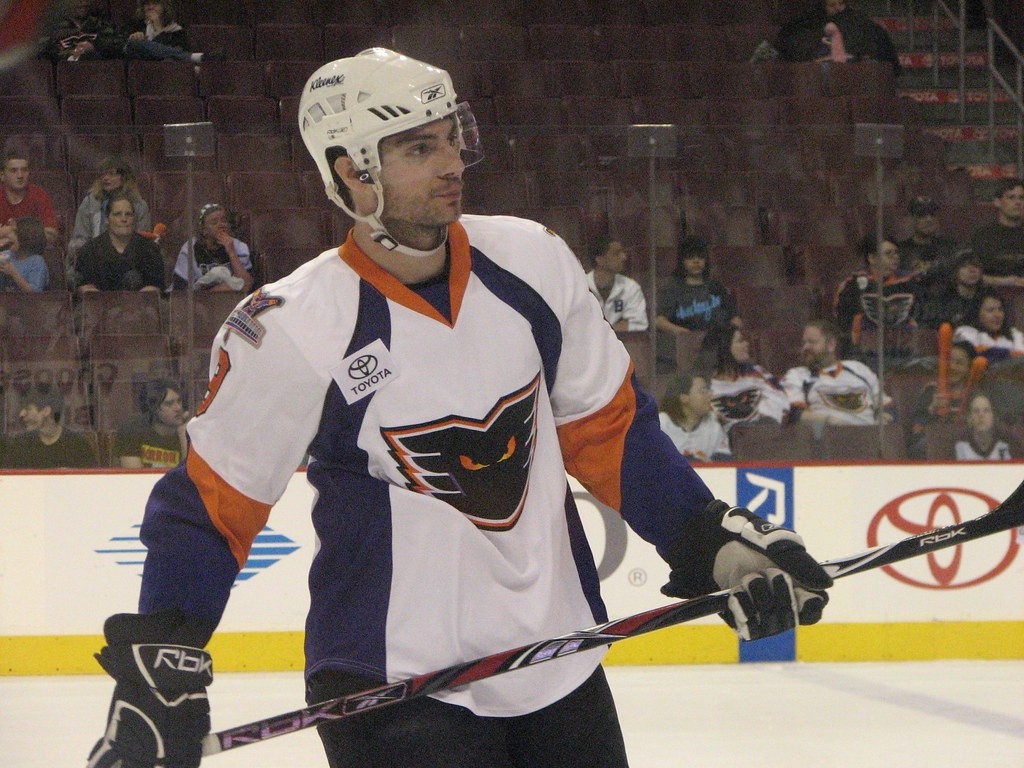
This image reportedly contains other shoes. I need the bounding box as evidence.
[202,48,229,61]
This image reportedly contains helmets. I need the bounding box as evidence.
[298,47,458,221]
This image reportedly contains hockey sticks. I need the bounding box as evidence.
[199,481,1024,757]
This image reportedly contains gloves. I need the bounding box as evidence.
[85,613,213,768]
[660,499,833,641]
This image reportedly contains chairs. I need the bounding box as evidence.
[0,0,1024,471]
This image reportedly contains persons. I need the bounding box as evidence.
[31,0,208,63]
[773,0,903,79]
[113,377,191,468]
[784,318,896,428]
[0,382,97,469]
[834,197,1024,365]
[658,372,734,464]
[972,177,1024,288]
[585,235,649,332]
[912,340,1012,461]
[688,323,789,427]
[656,237,738,375]
[83,47,833,768]
[0,153,164,292]
[172,202,254,294]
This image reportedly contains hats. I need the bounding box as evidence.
[196,202,225,225]
[908,195,941,213]
